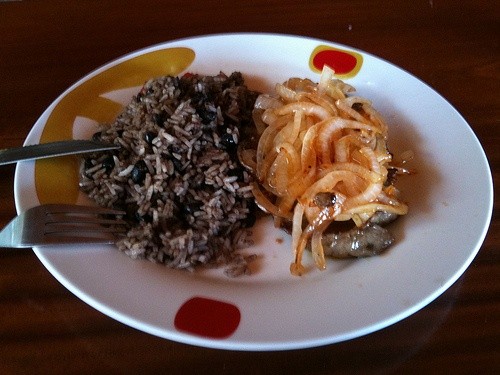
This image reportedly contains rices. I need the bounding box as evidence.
[77,74,258,280]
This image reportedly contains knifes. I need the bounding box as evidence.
[0,139,121,166]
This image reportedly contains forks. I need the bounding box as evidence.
[0,203,129,247]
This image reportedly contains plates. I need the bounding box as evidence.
[13,32,493,350]
